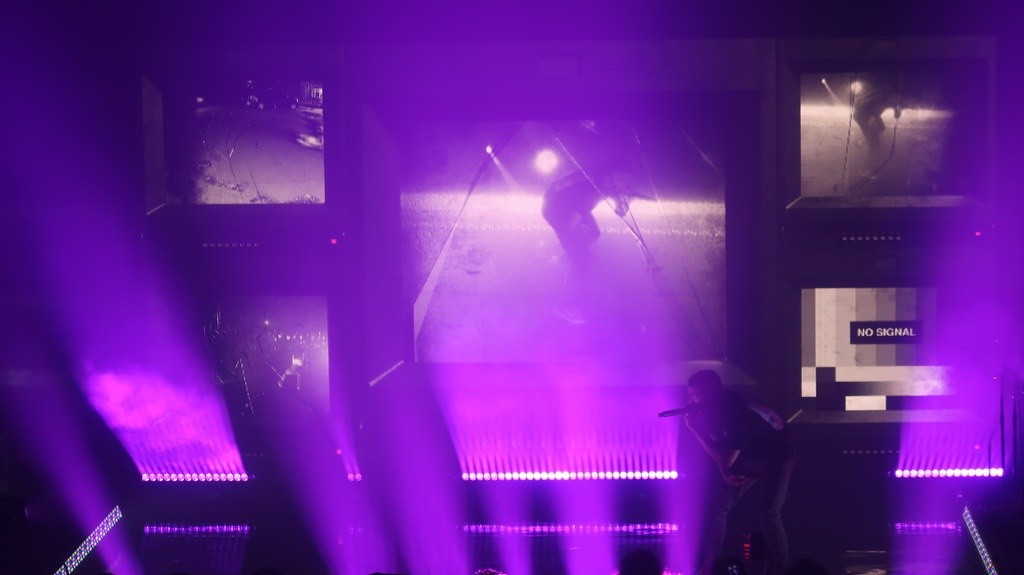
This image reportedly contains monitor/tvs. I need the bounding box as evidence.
[783,59,991,249]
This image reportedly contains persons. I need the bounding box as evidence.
[683,369,789,575]
[620,549,661,575]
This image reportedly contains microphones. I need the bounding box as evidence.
[658,406,689,417]
